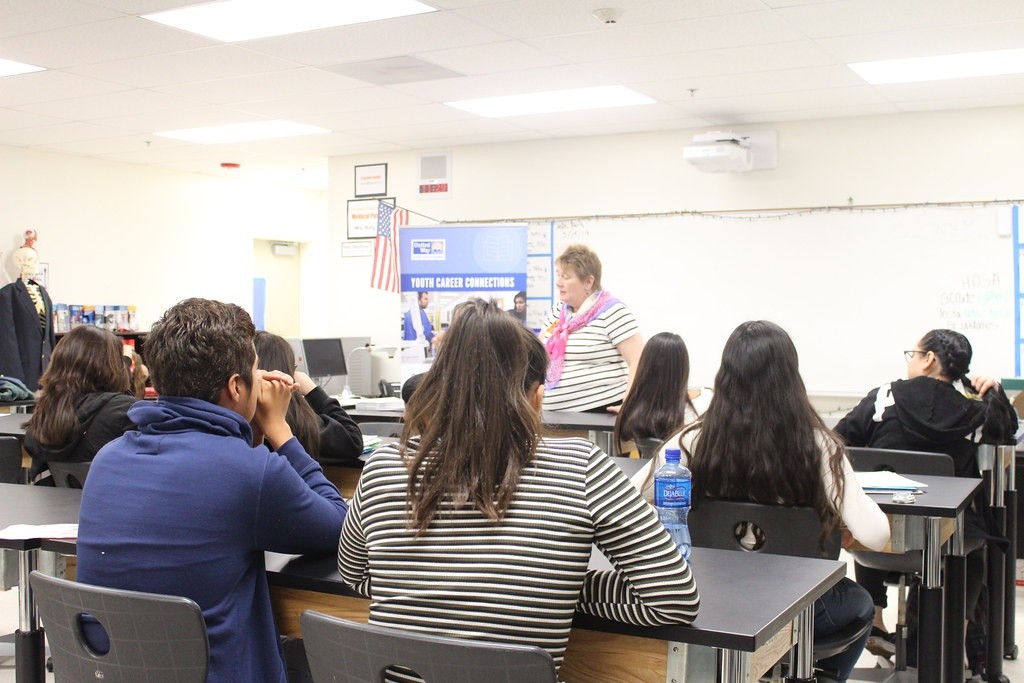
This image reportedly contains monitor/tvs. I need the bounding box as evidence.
[300,338,348,377]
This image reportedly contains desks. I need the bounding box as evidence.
[335,399,409,425]
[266,538,847,683]
[0,413,37,484]
[535,406,617,454]
[604,455,987,683]
[0,483,85,683]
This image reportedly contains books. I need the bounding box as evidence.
[122,340,136,368]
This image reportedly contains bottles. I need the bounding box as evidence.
[653,449,691,561]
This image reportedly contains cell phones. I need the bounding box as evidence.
[892,492,916,504]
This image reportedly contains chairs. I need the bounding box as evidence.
[0,370,987,683]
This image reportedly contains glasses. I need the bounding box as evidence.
[902,350,938,363]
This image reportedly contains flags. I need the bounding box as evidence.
[371,203,408,292]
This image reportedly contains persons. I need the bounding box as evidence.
[122,344,148,391]
[614,332,697,458]
[832,329,1019,654]
[514,291,526,318]
[630,321,891,683]
[403,291,433,345]
[538,245,644,413]
[75,299,347,683]
[401,373,430,434]
[336,299,699,683]
[23,326,141,490]
[253,330,364,462]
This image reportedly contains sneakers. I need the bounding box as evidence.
[866,626,897,658]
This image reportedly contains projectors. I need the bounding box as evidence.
[682,146,753,172]
